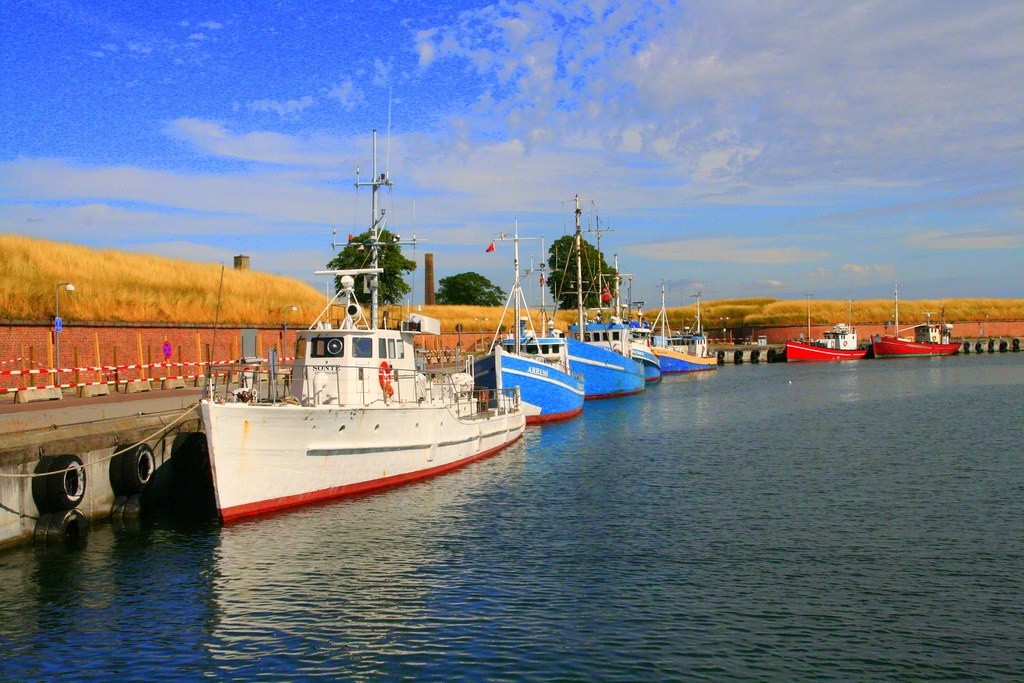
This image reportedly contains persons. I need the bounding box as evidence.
[444,346,452,363]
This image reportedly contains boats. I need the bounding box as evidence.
[485,193,720,423]
[784,323,868,363]
[196,130,526,523]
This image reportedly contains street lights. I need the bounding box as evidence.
[56,282,75,383]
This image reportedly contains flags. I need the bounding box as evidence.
[486,242,495,253]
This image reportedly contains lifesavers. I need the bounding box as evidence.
[46,455,86,507]
[171,432,209,476]
[1000,342,1007,352]
[124,442,156,488]
[843,341,847,346]
[932,336,936,341]
[976,342,983,353]
[111,491,161,521]
[378,361,391,389]
[964,342,970,354]
[1013,338,1019,352]
[988,340,994,353]
[718,348,777,366]
[34,509,87,544]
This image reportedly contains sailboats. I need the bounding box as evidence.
[871,311,962,359]
